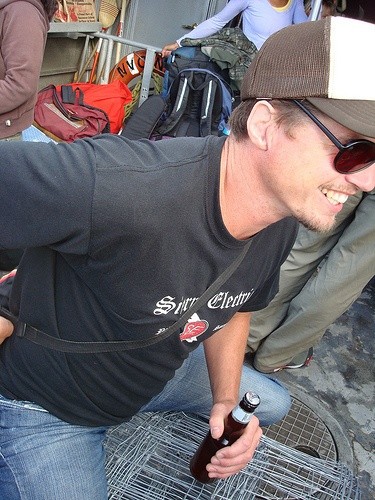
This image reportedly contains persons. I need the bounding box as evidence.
[161,0,375,372]
[0,1,58,144]
[0,15,375,500]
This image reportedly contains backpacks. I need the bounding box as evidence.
[119,26,259,140]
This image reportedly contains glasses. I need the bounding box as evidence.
[293,99,375,174]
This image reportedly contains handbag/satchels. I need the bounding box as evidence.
[34,83,110,143]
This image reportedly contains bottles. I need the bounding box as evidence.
[189,392,261,485]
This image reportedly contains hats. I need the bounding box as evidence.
[240,15,375,138]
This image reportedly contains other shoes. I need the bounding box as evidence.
[252,346,314,374]
